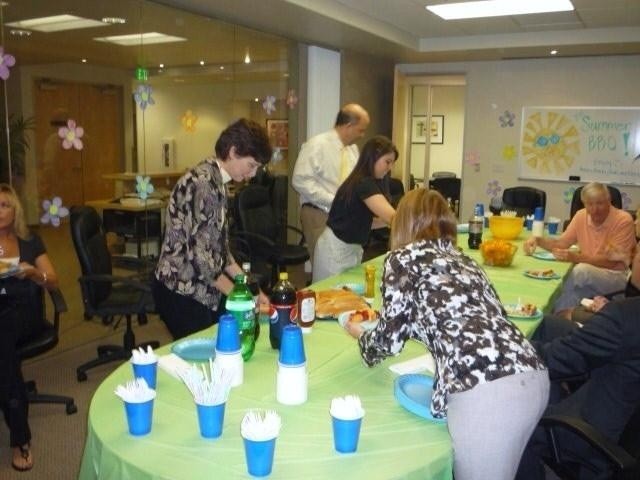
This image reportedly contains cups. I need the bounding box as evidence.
[278,325,307,367]
[534,207,542,221]
[194,400,225,440]
[131,358,158,391]
[549,222,558,235]
[526,219,533,231]
[240,426,277,477]
[476,203,484,216]
[215,315,242,356]
[327,409,363,454]
[123,399,155,437]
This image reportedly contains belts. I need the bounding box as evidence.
[303,202,325,210]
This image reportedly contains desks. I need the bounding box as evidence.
[84,192,169,276]
[102,169,188,198]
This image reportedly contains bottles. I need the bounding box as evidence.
[240,262,261,342]
[469,207,485,251]
[269,272,300,350]
[365,266,376,298]
[297,290,317,334]
[226,273,257,363]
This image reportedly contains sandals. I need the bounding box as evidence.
[12,442,33,472]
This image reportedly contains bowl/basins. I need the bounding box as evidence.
[479,239,519,267]
[489,216,523,240]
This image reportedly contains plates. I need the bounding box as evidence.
[336,282,367,296]
[503,301,543,320]
[0,260,20,279]
[523,267,561,280]
[314,285,372,321]
[533,250,557,260]
[170,334,218,364]
[395,371,446,425]
[338,309,379,330]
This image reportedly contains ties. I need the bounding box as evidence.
[339,145,351,182]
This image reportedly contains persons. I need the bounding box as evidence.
[313,136,398,280]
[0,183,59,473]
[348,189,550,480]
[154,119,272,341]
[529,237,640,466]
[293,104,371,265]
[524,182,636,324]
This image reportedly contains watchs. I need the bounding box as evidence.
[37,273,47,286]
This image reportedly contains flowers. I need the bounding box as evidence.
[271,148,283,166]
[487,181,501,197]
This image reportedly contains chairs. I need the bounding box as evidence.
[538,416,639,480]
[70,205,160,382]
[231,185,311,289]
[563,184,623,233]
[17,280,78,415]
[433,172,455,178]
[429,178,461,218]
[490,187,547,226]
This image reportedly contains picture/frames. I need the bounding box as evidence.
[411,115,444,145]
[265,118,288,151]
